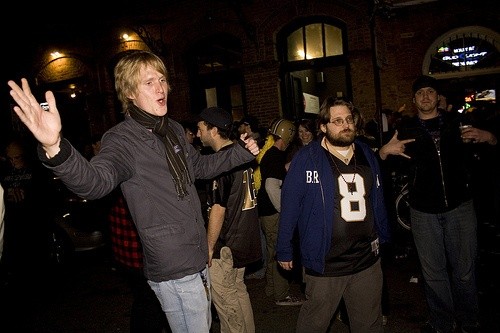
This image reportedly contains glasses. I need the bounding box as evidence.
[329,117,354,126]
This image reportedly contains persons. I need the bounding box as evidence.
[8,51,260,333]
[0,74,500,333]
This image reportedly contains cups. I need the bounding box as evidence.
[458,122,473,143]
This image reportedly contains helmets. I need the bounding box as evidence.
[271,120,295,145]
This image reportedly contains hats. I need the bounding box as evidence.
[413,75,439,97]
[200,106,233,132]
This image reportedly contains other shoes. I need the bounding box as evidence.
[430,320,456,333]
[275,295,303,305]
[461,318,480,333]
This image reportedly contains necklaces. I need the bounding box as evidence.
[324,136,357,195]
